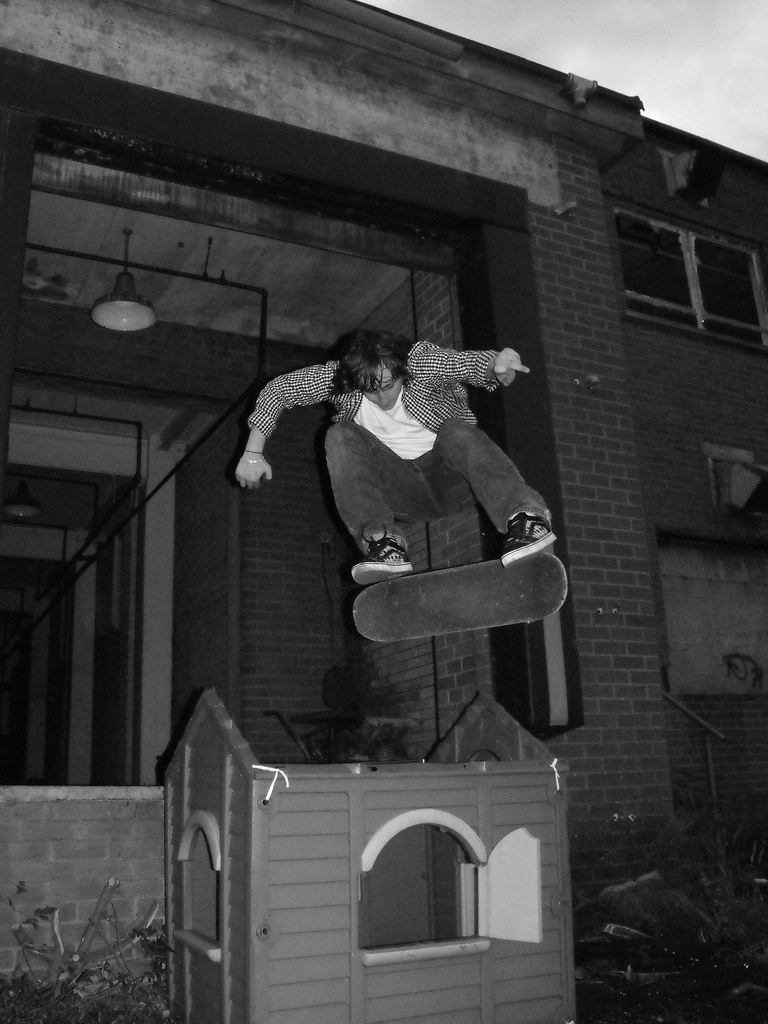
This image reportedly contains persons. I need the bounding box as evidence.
[233,329,558,584]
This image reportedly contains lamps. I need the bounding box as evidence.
[89,229,158,332]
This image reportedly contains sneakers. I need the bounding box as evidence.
[501,512,556,568]
[351,522,413,585]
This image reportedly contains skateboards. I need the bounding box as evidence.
[351,550,572,645]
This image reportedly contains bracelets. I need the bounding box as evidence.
[245,450,264,454]
[239,456,265,464]
